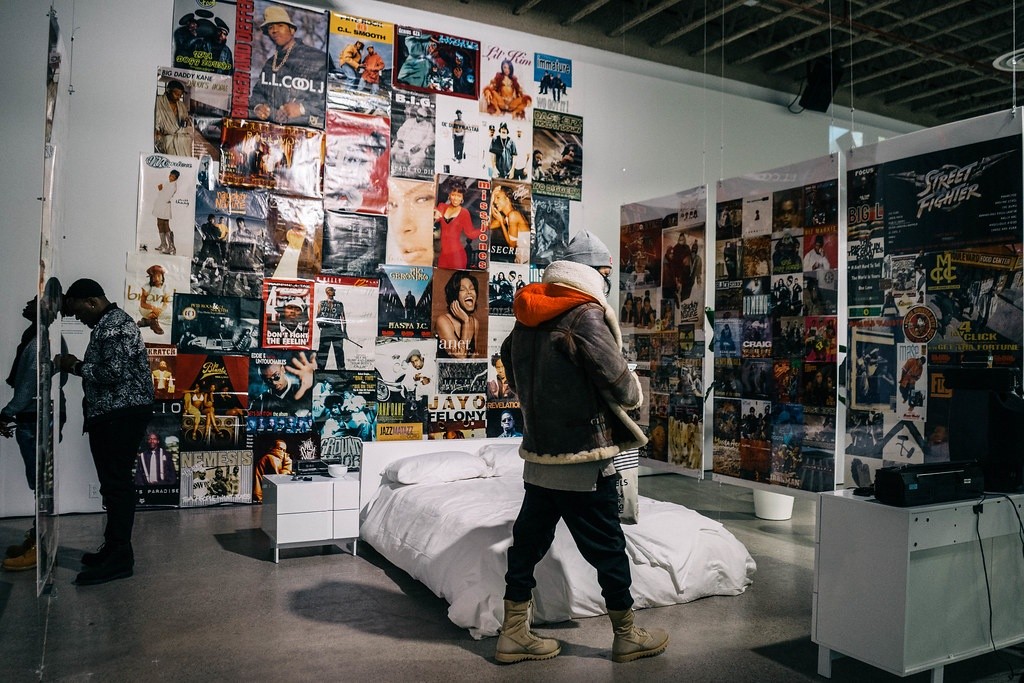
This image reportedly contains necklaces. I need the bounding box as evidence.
[272,42,295,72]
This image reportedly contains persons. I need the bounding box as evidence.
[157,6,582,501]
[152,169,180,255]
[496,228,671,664]
[60,279,156,586]
[137,265,169,335]
[619,197,1024,492]
[134,361,245,504]
[0,277,60,569]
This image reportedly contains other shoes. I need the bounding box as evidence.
[3,545,36,570]
[151,317,164,334]
[7,529,36,556]
[137,318,150,327]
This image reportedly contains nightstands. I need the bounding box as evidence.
[262,474,359,564]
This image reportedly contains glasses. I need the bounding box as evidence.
[264,369,281,384]
[501,417,512,422]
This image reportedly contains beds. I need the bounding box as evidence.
[359,437,756,642]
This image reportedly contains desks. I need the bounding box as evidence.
[811,485,1024,683]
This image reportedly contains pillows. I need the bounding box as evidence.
[478,444,526,477]
[380,451,492,484]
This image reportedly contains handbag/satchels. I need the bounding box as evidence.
[613,444,640,525]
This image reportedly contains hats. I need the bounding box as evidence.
[259,5,298,36]
[274,295,306,315]
[498,123,509,133]
[147,265,165,275]
[405,349,421,363]
[563,229,612,268]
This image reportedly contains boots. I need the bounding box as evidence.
[494,598,562,663]
[606,606,669,662]
[162,231,176,255]
[76,510,135,586]
[156,232,167,250]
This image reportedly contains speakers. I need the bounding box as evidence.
[799,55,845,112]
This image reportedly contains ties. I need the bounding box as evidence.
[150,451,157,483]
